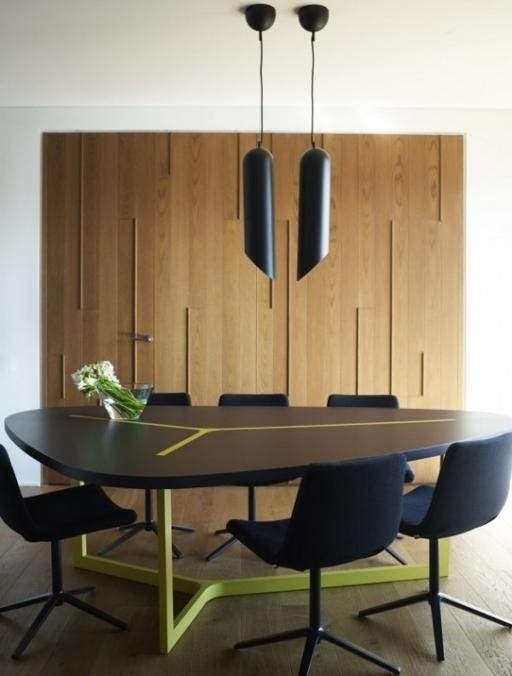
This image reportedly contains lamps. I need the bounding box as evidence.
[295,4,337,282]
[241,5,284,282]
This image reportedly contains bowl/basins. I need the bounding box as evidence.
[96,383,155,422]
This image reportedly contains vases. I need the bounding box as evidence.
[103,384,156,420]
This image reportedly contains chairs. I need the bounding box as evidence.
[357,432,512,663]
[326,391,410,566]
[1,446,125,661]
[225,450,408,674]
[98,391,197,561]
[206,392,294,568]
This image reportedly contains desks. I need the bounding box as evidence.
[2,402,512,657]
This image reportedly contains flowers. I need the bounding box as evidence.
[66,359,147,416]
